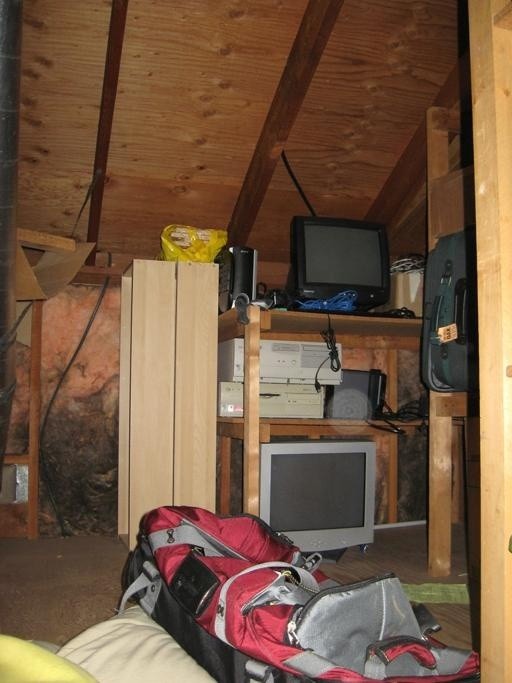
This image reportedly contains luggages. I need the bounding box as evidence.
[421,230,478,393]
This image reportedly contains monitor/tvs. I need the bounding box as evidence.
[260,441,376,553]
[290,215,390,312]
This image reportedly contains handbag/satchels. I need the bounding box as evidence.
[121,507,480,682]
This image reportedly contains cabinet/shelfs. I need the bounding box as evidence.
[218,308,429,525]
